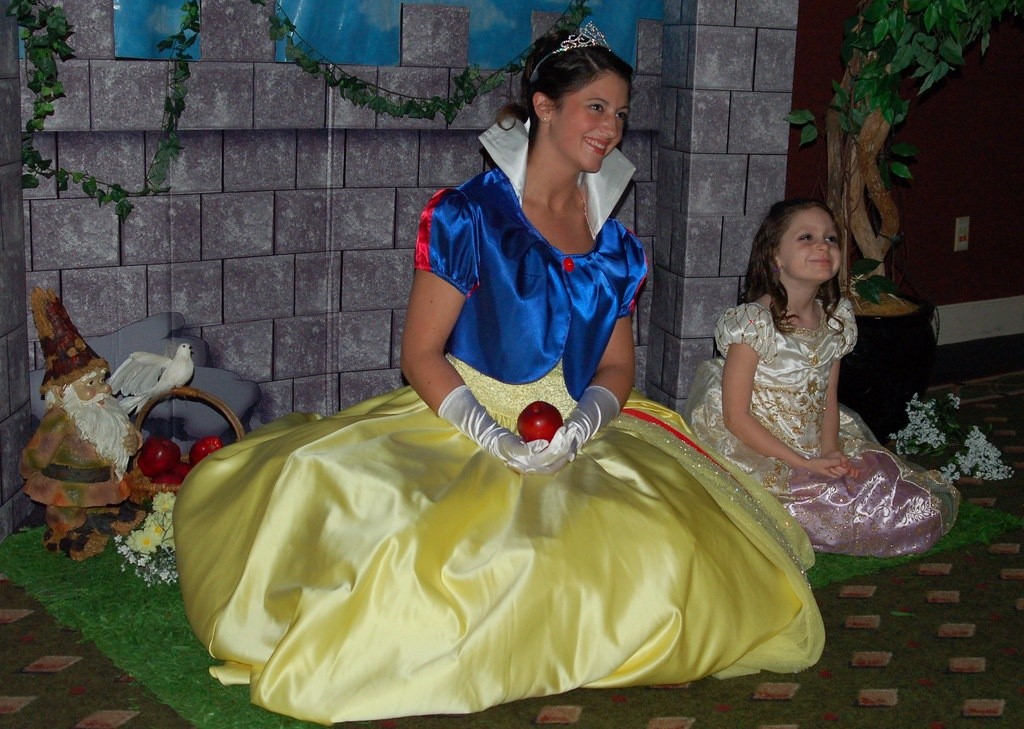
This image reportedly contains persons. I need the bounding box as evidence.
[684,197,961,558]
[19,286,147,562]
[172,19,824,726]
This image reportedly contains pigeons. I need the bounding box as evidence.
[108,343,195,415]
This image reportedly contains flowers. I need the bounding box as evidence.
[111,492,179,586]
[888,390,1015,484]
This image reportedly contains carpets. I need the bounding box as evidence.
[1,499,1024,729]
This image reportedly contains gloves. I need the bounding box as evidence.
[438,385,578,468]
[503,384,621,475]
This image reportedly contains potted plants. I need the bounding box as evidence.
[782,0,1024,442]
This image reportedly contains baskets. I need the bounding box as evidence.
[126,388,247,506]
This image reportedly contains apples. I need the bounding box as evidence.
[139,434,223,484]
[516,400,563,445]
